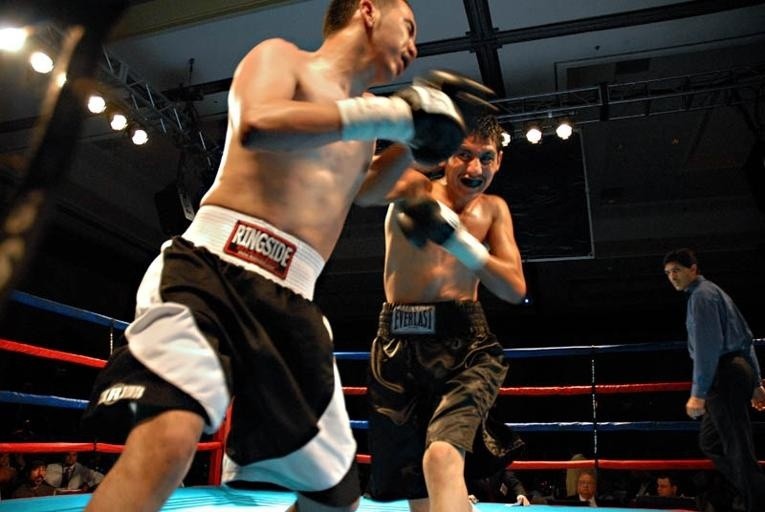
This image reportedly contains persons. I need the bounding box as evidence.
[662,246,765,511]
[353,105,530,505]
[515,450,680,510]
[1,450,107,502]
[74,0,501,511]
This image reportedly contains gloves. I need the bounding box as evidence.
[391,195,489,268]
[333,67,498,166]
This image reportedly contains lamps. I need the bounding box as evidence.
[0,9,149,147]
[501,113,576,149]
